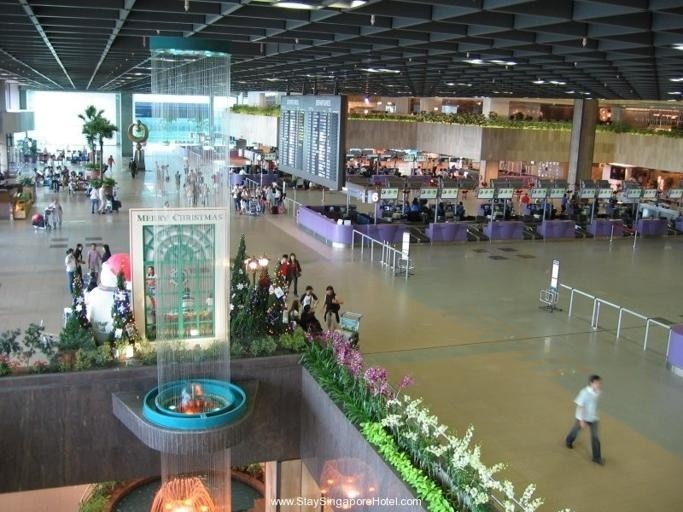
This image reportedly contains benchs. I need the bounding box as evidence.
[32,150,96,197]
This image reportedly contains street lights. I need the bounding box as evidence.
[241,252,269,290]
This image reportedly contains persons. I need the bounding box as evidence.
[183,288,194,337]
[346,160,473,223]
[129,158,137,179]
[565,374,604,466]
[155,159,287,217]
[64,242,112,295]
[48,199,63,231]
[507,189,654,229]
[145,266,158,310]
[32,147,122,214]
[205,293,214,312]
[276,253,343,335]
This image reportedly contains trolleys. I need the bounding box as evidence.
[338,311,363,351]
[244,197,257,216]
[105,194,119,213]
[32,209,52,232]
